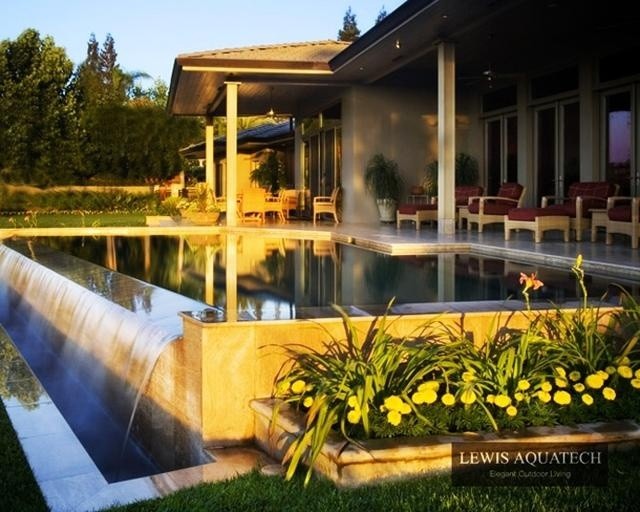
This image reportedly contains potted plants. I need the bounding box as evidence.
[362,151,407,222]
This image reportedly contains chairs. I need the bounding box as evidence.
[208,187,301,223]
[395,180,640,250]
[312,184,341,226]
